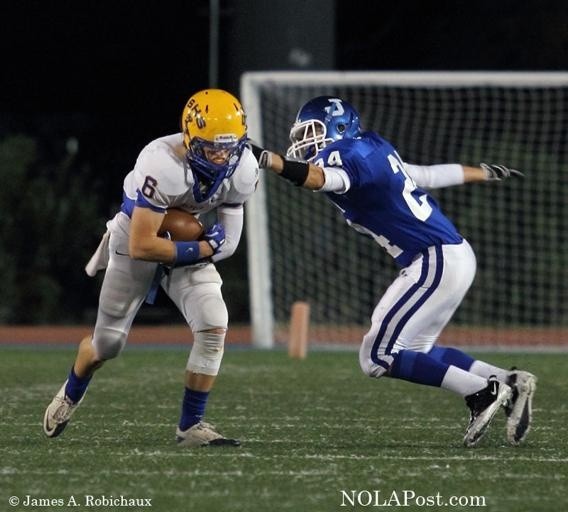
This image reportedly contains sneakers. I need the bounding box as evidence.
[42,378,88,437]
[504,368,537,446]
[463,376,513,448]
[175,421,240,447]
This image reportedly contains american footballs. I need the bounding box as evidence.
[158,207,205,241]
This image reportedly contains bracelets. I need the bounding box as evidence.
[277,154,312,189]
[173,240,201,268]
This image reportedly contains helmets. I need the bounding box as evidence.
[287,95,361,162]
[182,89,247,182]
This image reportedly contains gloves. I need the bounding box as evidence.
[201,222,226,253]
[481,162,526,182]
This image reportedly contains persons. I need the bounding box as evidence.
[241,95,538,449]
[43,90,264,449]
